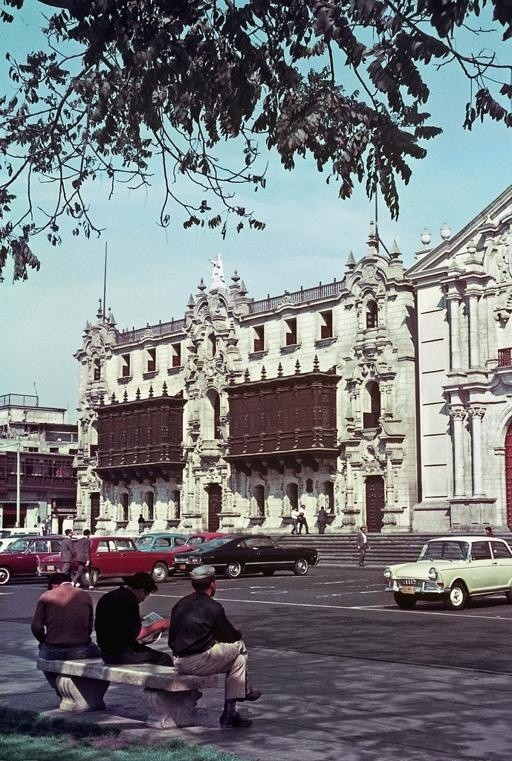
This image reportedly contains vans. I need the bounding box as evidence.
[0,538,50,554]
[0,528,43,536]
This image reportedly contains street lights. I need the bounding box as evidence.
[5,428,29,527]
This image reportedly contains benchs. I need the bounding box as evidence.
[36,655,219,730]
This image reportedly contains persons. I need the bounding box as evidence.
[139,514,145,533]
[71,529,95,589]
[61,529,77,574]
[318,506,328,534]
[206,252,228,289]
[95,572,203,705]
[31,573,100,701]
[291,507,300,535]
[42,514,54,534]
[357,527,368,567]
[298,505,310,534]
[168,565,261,728]
[485,527,495,549]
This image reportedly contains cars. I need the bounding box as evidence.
[122,532,188,551]
[34,537,174,586]
[0,537,73,585]
[383,536,512,610]
[173,534,319,577]
[167,533,248,577]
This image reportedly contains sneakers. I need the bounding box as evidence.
[237,684,261,702]
[220,713,253,728]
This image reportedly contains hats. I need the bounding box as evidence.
[189,564,215,582]
[122,572,153,592]
